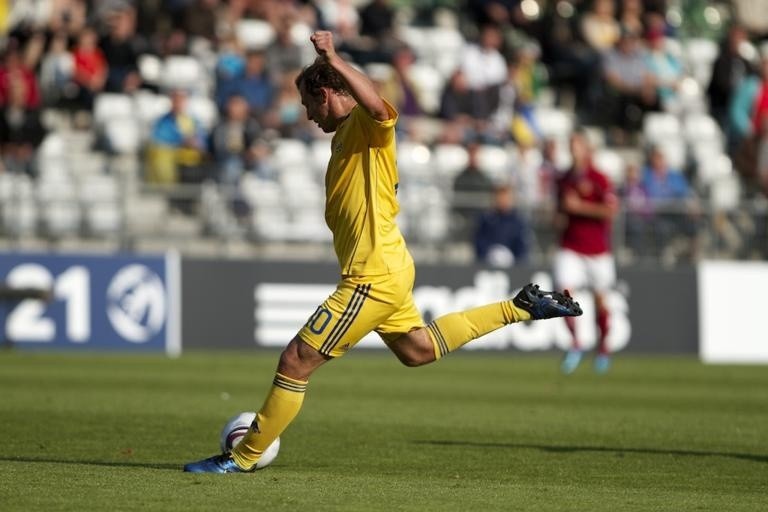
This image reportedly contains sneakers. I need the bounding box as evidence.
[183,453,257,472]
[513,283,583,320]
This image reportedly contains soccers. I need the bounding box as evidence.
[220,412,280,469]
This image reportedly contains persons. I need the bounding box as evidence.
[0,0,767,268]
[550,134,619,376]
[183,30,583,474]
